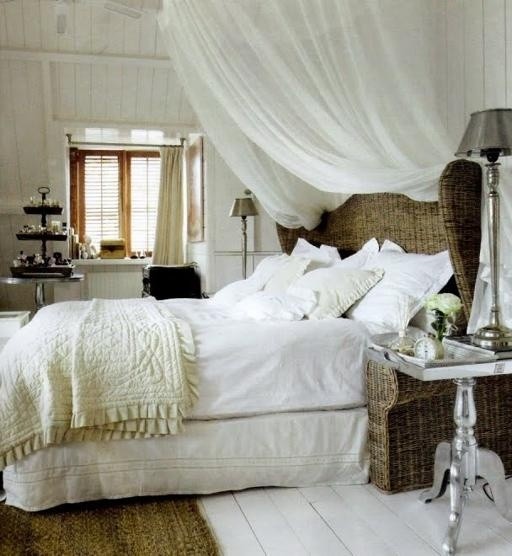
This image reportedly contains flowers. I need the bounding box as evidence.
[425,292,465,340]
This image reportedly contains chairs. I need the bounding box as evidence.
[141,266,202,299]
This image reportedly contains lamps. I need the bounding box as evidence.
[229,198,259,280]
[454,107,512,352]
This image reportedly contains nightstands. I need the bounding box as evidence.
[367,344,512,556]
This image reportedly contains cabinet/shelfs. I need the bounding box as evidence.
[9,186,77,277]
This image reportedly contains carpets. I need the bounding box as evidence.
[0,494,224,556]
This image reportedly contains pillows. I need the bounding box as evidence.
[347,239,452,336]
[291,265,385,321]
[230,283,319,323]
[319,243,342,263]
[290,238,334,273]
[208,277,264,308]
[411,299,454,335]
[254,252,311,298]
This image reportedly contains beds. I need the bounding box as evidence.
[0,159,512,511]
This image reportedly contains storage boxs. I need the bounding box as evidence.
[100,238,127,259]
[0,310,32,353]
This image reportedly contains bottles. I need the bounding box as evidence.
[11,249,73,267]
[26,195,63,206]
[22,220,68,237]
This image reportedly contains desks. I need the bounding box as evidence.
[0,273,86,315]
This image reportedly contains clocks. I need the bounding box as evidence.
[414,334,444,361]
